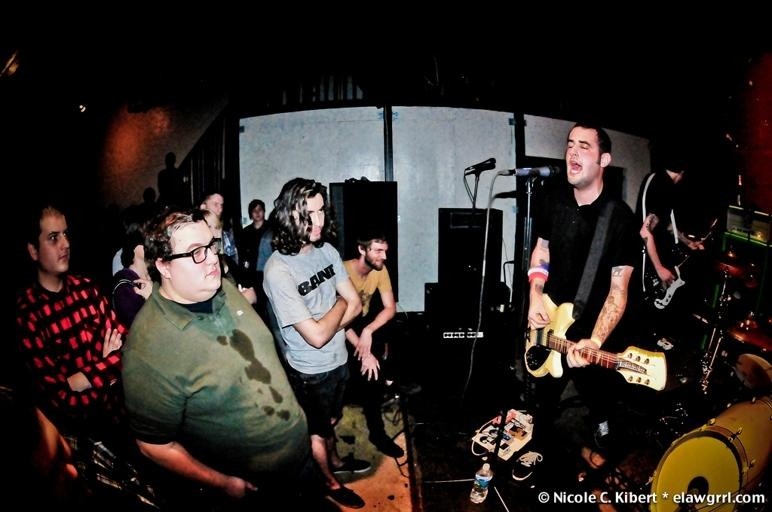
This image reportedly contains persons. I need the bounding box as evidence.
[238,223,282,321]
[242,199,267,260]
[198,189,238,264]
[12,205,128,456]
[511,124,643,479]
[156,151,182,212]
[634,147,703,329]
[137,186,157,223]
[110,228,152,330]
[112,199,171,276]
[122,212,325,512]
[341,227,404,457]
[263,178,371,509]
[0,378,74,510]
[194,209,223,238]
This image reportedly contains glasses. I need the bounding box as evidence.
[163,237,222,264]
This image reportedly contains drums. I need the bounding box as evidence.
[707,250,772,368]
[715,354,772,408]
[650,397,772,512]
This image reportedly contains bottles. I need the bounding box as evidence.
[471,463,492,504]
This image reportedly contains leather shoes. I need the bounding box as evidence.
[333,451,372,475]
[327,483,367,509]
[368,431,405,459]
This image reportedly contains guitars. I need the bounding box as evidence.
[525,294,666,390]
[641,232,714,310]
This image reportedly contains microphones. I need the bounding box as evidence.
[464,159,495,177]
[494,166,560,177]
[497,190,538,198]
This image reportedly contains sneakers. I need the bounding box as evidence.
[512,450,543,481]
